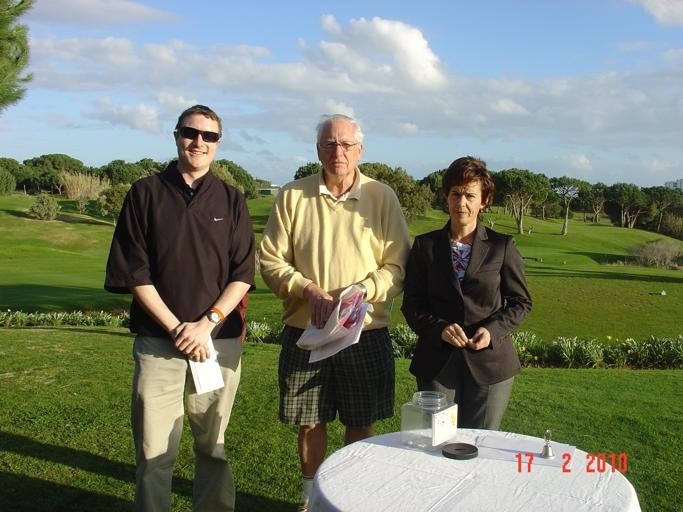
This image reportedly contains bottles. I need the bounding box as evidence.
[538,429,556,460]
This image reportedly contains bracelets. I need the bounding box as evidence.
[356,283,368,298]
[209,306,225,322]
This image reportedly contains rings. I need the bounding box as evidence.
[450,336,454,340]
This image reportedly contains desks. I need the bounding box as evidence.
[307,426,647,512]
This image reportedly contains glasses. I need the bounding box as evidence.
[316,141,358,149]
[177,125,222,143]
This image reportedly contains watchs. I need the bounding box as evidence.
[205,311,223,328]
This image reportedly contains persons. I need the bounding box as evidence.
[399,155,533,431]
[103,104,255,512]
[258,113,411,512]
